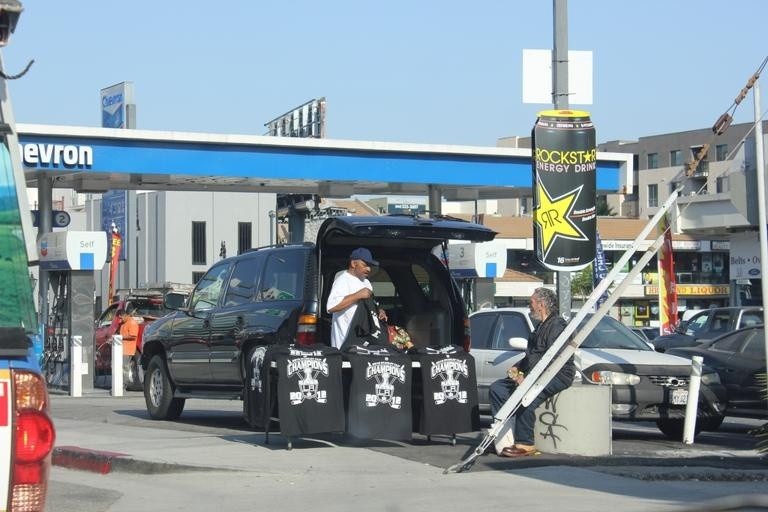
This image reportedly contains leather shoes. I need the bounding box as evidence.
[503,442,536,457]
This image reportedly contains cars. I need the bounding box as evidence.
[466,307,727,438]
[631,305,767,430]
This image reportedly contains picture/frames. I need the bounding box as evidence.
[634,300,650,319]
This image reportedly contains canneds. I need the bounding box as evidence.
[531,109,597,272]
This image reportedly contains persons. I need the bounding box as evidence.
[326,248,388,355]
[490,288,574,456]
[115,308,138,392]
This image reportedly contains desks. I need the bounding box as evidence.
[265,361,456,450]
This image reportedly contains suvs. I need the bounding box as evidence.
[93,297,171,390]
[140,216,499,419]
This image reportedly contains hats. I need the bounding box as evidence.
[351,247,380,265]
